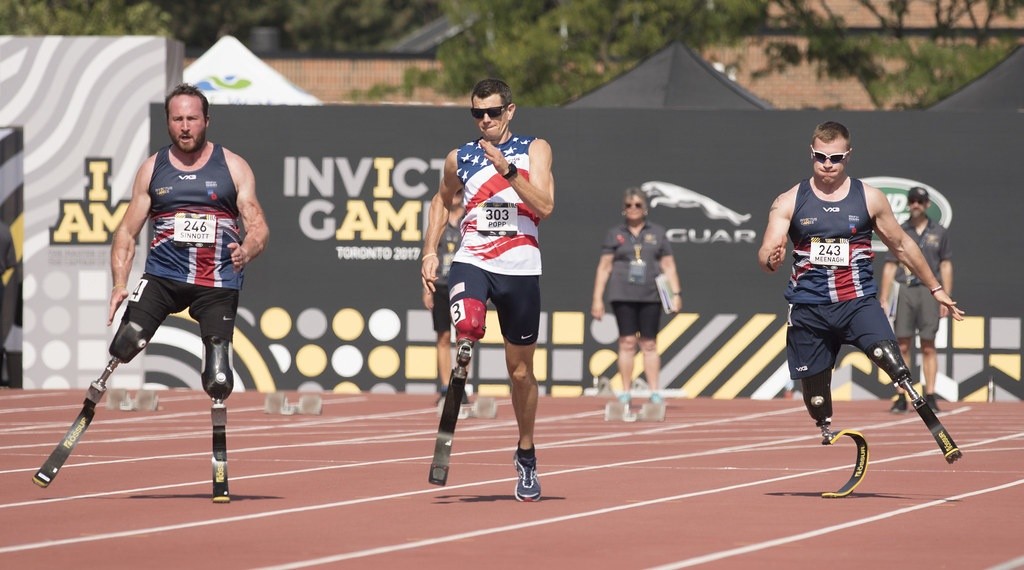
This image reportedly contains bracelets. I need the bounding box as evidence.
[673,292,682,296]
[423,253,437,261]
[932,286,942,294]
[111,284,125,292]
[767,256,775,272]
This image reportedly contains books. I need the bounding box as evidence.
[654,274,675,314]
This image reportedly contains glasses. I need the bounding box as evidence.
[625,203,645,209]
[470,103,507,119]
[811,145,852,164]
[910,199,925,204]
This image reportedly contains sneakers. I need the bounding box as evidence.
[513,452,541,503]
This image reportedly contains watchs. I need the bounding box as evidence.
[502,162,518,179]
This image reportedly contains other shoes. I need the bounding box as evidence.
[928,402,939,413]
[891,400,908,414]
[651,393,663,403]
[619,393,631,404]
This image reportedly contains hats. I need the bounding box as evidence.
[908,187,928,202]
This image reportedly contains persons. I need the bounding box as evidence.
[421,79,555,503]
[758,121,965,497]
[880,188,953,413]
[30,83,270,502]
[591,187,683,406]
